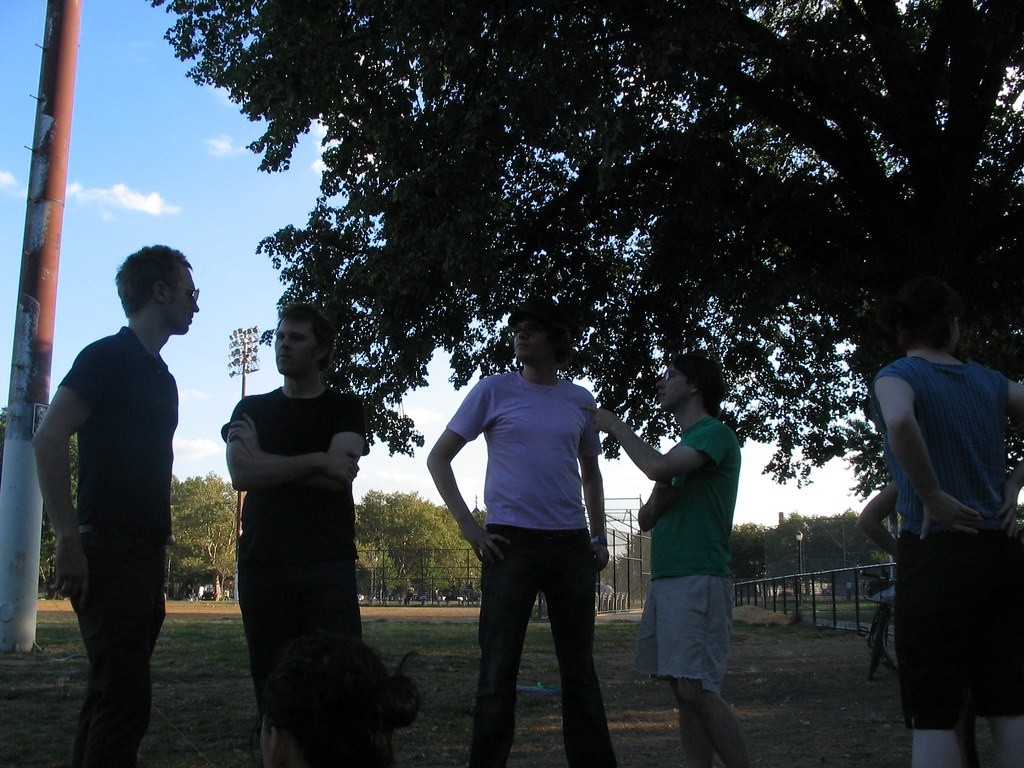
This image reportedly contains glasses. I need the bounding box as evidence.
[170,286,199,303]
[664,370,686,381]
[510,326,546,337]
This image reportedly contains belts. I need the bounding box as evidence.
[78,524,95,534]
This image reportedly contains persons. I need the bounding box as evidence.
[32,245,199,768]
[427,298,618,768]
[579,353,751,768]
[248,630,421,768]
[434,588,438,601]
[464,582,473,593]
[221,302,370,740]
[224,588,229,600]
[857,481,898,555]
[870,278,1024,767]
[198,585,205,599]
[404,585,414,604]
[602,582,614,594]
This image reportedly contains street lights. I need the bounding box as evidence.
[228,324,260,602]
[795,529,804,605]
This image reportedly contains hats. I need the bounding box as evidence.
[508,298,567,329]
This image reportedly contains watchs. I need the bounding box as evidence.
[591,536,607,546]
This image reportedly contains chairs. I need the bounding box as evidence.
[596,591,629,610]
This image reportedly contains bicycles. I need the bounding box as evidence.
[856,563,900,679]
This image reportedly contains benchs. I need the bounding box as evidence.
[435,589,482,606]
[399,599,428,607]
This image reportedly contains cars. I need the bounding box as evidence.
[201,591,216,601]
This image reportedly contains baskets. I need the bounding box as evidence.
[860,579,897,605]
